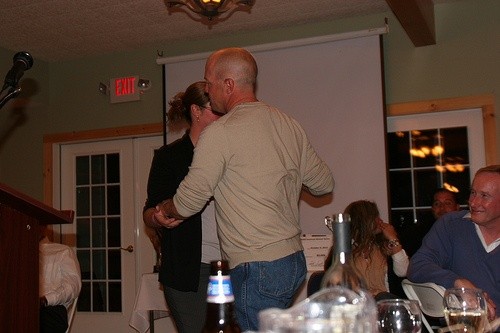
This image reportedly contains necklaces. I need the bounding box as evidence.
[360,249,373,264]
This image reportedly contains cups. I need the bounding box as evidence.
[442,287,488,333]
[375,298,422,333]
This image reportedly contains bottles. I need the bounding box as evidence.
[200,260,242,333]
[320,212,367,303]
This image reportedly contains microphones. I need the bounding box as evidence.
[2,51,34,90]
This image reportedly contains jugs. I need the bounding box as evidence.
[258,288,377,333]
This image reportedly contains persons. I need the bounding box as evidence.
[406,165,500,333]
[38,224,82,333]
[431,189,459,220]
[143,78,222,333]
[325,200,409,303]
[162,47,334,331]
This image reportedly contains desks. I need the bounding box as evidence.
[129,274,169,333]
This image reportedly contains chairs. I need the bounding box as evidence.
[402,279,462,333]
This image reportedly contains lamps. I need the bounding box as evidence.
[98,75,152,104]
[163,0,255,22]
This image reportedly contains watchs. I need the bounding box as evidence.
[39,297,45,307]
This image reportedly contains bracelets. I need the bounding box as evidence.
[387,240,399,248]
